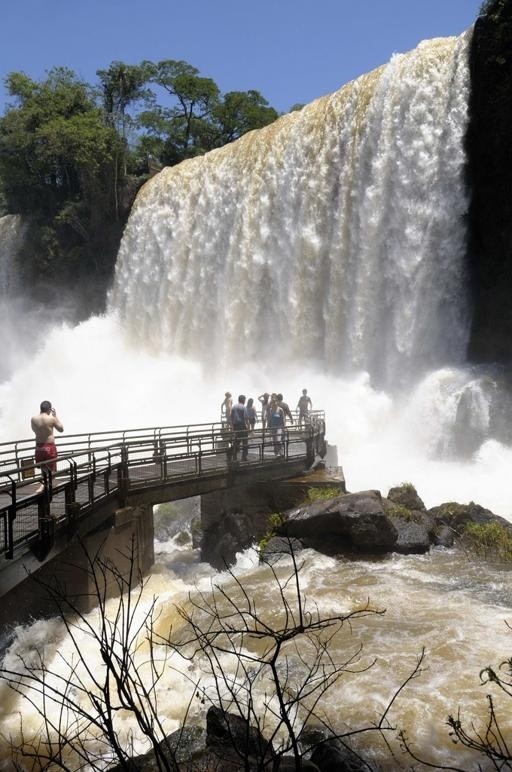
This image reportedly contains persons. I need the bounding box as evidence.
[30,399,63,496]
[220,389,313,464]
